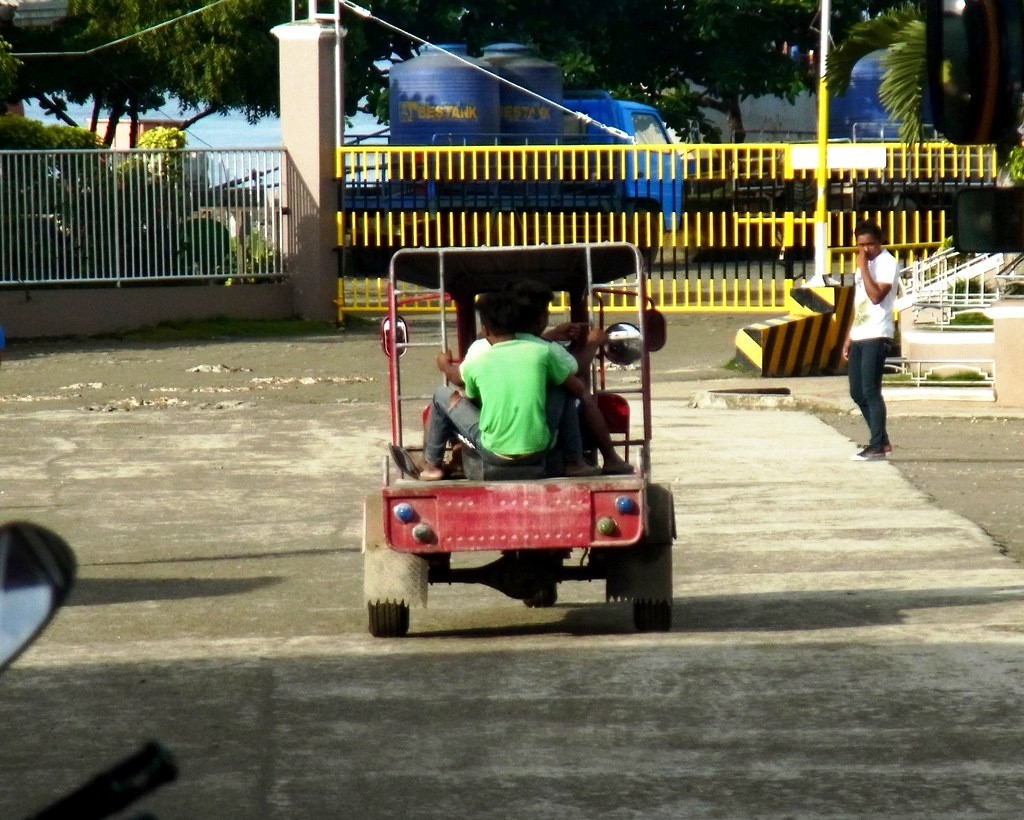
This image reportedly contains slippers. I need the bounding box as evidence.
[389,443,425,480]
[603,458,634,473]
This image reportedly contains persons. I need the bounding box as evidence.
[842,220,901,460]
[416,286,634,479]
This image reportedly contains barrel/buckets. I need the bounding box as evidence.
[829,96,849,139]
[388,44,502,166]
[847,47,933,140]
[480,41,565,145]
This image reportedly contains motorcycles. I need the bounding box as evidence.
[0,520,180,820]
[362,242,673,638]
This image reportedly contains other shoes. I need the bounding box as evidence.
[417,469,445,481]
[858,444,892,455]
[446,455,463,471]
[565,463,602,475]
[852,446,886,461]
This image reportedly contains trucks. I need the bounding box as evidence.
[346,91,684,270]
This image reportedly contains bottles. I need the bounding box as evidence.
[556,326,576,351]
